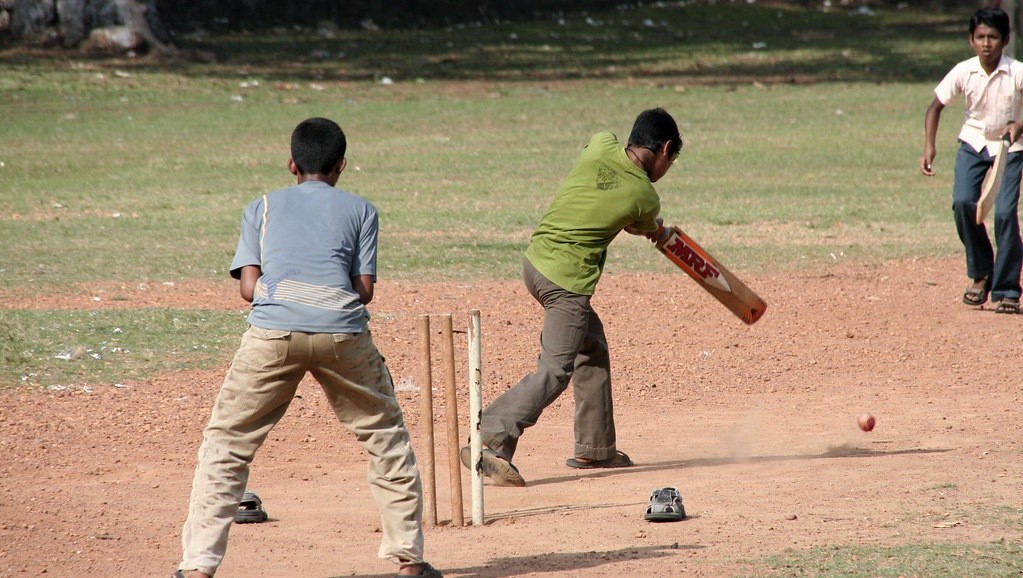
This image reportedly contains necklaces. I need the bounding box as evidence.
[626,147,645,167]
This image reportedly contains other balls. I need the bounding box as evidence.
[858,414,876,433]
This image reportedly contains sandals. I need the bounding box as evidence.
[235,493,267,523]
[962,271,993,305]
[996,297,1020,313]
[644,487,684,519]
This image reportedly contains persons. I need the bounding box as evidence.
[920,7,1023,315]
[173,117,443,578]
[461,107,682,489]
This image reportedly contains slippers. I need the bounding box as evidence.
[171,569,186,578]
[461,444,525,487]
[396,562,443,578]
[566,448,633,468]
